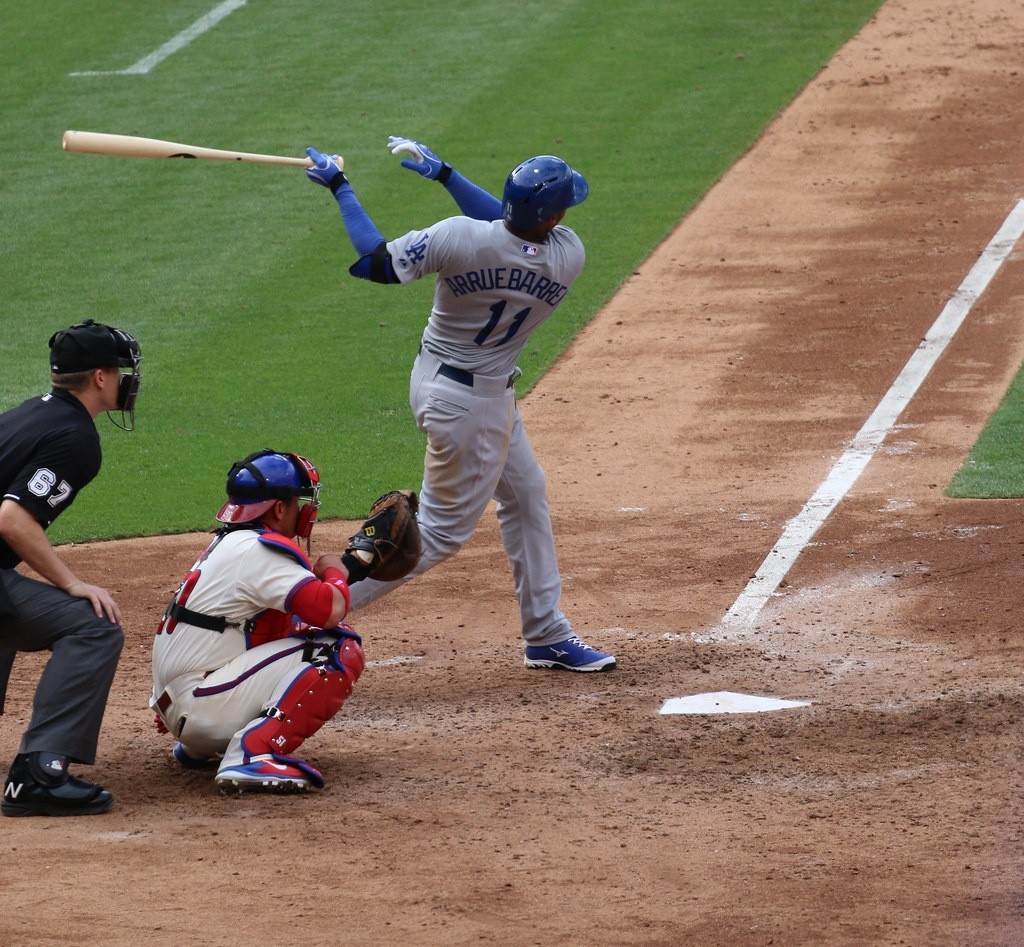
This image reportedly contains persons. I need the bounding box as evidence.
[305,135,618,672]
[149,448,421,795]
[0,317,142,816]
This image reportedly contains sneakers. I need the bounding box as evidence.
[173,742,224,772]
[1,751,113,816]
[525,636,616,673]
[214,756,311,794]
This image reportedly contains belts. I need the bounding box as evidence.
[418,345,516,388]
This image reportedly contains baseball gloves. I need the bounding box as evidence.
[342,489,424,588]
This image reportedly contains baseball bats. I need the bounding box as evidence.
[62,129,347,174]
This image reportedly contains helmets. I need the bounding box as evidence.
[215,448,313,523]
[501,156,591,227]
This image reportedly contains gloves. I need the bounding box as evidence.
[305,147,351,196]
[387,135,452,187]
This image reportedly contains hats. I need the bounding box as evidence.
[49,320,136,374]
